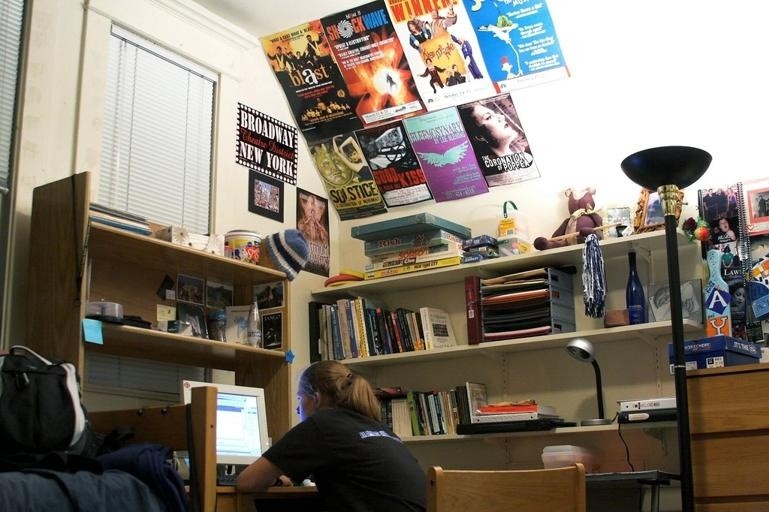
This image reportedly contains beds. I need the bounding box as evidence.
[0,385,219,512]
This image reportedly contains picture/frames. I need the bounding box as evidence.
[248,168,284,222]
[741,176,769,237]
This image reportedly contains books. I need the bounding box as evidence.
[366,232,461,280]
[308,297,556,438]
[89,201,154,237]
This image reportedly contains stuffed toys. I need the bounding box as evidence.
[533,186,602,250]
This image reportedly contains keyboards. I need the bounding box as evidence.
[215,473,239,486]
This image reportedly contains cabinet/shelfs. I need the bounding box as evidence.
[683,362,769,512]
[310,225,702,444]
[26,172,292,475]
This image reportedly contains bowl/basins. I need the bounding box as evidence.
[223,230,262,265]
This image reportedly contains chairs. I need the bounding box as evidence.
[423,461,588,512]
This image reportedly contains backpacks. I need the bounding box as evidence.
[0,345,86,474]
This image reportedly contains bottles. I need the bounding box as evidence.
[625,248,646,324]
[172,451,190,481]
[215,308,227,342]
[246,296,262,348]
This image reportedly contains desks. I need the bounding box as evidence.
[213,476,318,511]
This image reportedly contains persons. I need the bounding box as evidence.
[701,186,769,338]
[332,133,374,183]
[312,141,357,187]
[406,2,484,95]
[459,103,533,172]
[234,360,428,512]
[267,32,351,125]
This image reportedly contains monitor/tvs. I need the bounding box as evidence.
[180,379,269,474]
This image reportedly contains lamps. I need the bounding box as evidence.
[565,337,611,426]
[621,146,714,512]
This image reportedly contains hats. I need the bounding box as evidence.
[265,229,310,282]
